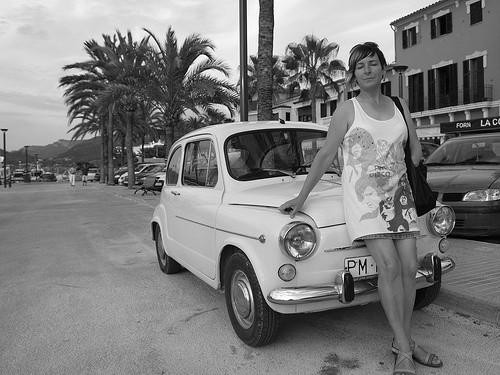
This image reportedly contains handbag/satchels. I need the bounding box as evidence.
[408,158,437,217]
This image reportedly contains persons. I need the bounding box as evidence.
[82,163,89,186]
[279,41,442,375]
[68,164,77,186]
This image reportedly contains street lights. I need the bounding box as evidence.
[23,145,30,174]
[0,128,8,187]
[32,153,38,180]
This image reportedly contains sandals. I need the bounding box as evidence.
[393,350,416,375]
[392,336,444,367]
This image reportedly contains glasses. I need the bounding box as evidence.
[349,42,377,55]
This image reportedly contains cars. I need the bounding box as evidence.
[425,116,500,240]
[149,120,457,347]
[87,168,102,182]
[63,169,83,181]
[42,173,58,182]
[114,162,167,191]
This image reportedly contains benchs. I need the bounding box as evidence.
[133,174,160,197]
